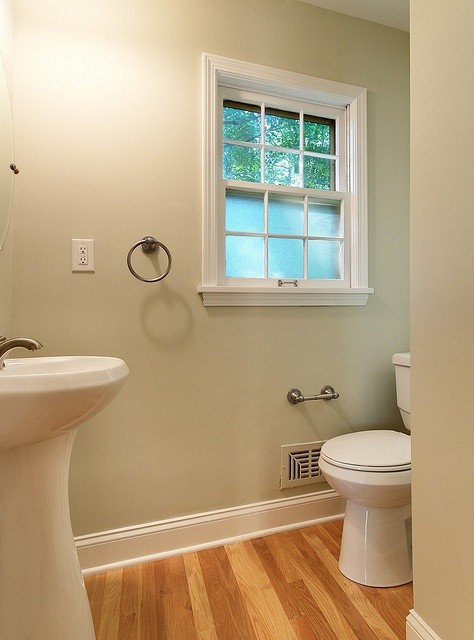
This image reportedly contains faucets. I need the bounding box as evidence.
[1,334,47,368]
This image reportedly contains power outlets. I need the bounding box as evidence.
[71,238,96,272]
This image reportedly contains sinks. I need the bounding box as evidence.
[0,356,130,450]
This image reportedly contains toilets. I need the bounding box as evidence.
[320,352,414,588]
